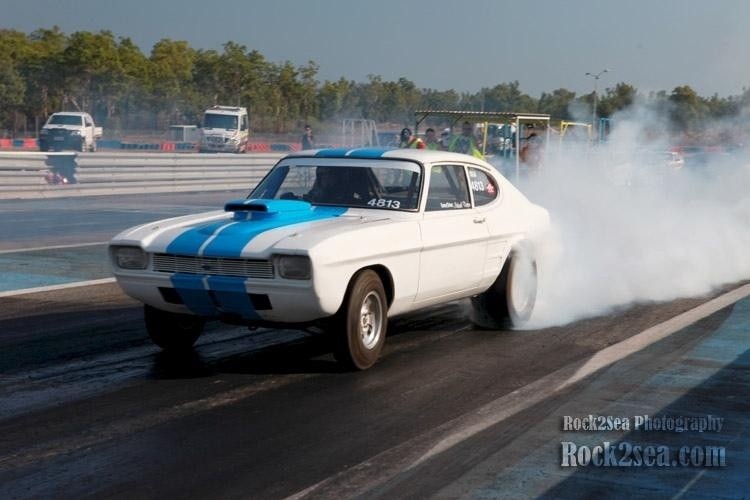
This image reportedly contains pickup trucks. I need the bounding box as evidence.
[38,109,104,154]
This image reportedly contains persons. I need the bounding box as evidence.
[389,121,545,186]
[279,166,362,204]
[301,124,315,149]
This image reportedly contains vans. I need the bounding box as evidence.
[198,105,251,154]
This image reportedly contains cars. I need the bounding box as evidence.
[102,141,556,374]
[647,150,686,173]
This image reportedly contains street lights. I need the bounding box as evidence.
[584,67,609,146]
